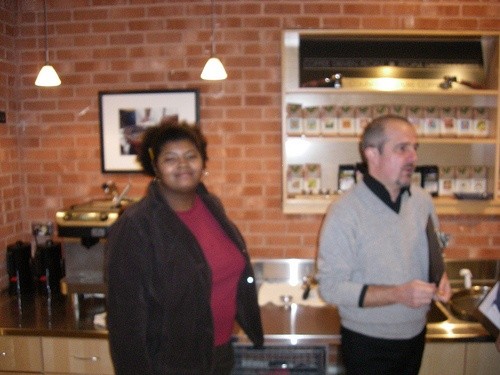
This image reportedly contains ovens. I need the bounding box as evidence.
[232,347,326,375]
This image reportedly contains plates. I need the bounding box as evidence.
[449,288,491,322]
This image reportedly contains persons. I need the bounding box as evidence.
[317,115,451,375]
[102,124,265,375]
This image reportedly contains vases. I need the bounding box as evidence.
[97,87,204,175]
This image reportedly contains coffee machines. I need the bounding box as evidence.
[53,200,132,319]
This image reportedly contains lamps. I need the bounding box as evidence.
[33,0,62,88]
[200,0,228,81]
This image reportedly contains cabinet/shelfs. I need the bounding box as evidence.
[0,277,500,375]
[281,27,500,216]
